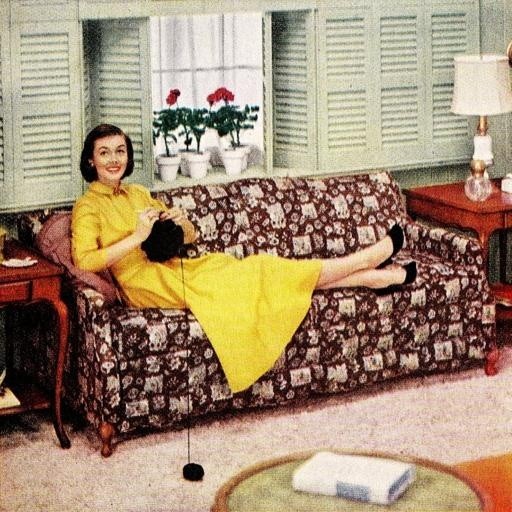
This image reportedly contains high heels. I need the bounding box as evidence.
[373,224,404,269]
[374,260,417,297]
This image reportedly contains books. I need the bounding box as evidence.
[290,450,419,505]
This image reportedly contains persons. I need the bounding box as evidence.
[70,123,418,395]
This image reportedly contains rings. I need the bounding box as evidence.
[148,214,153,221]
